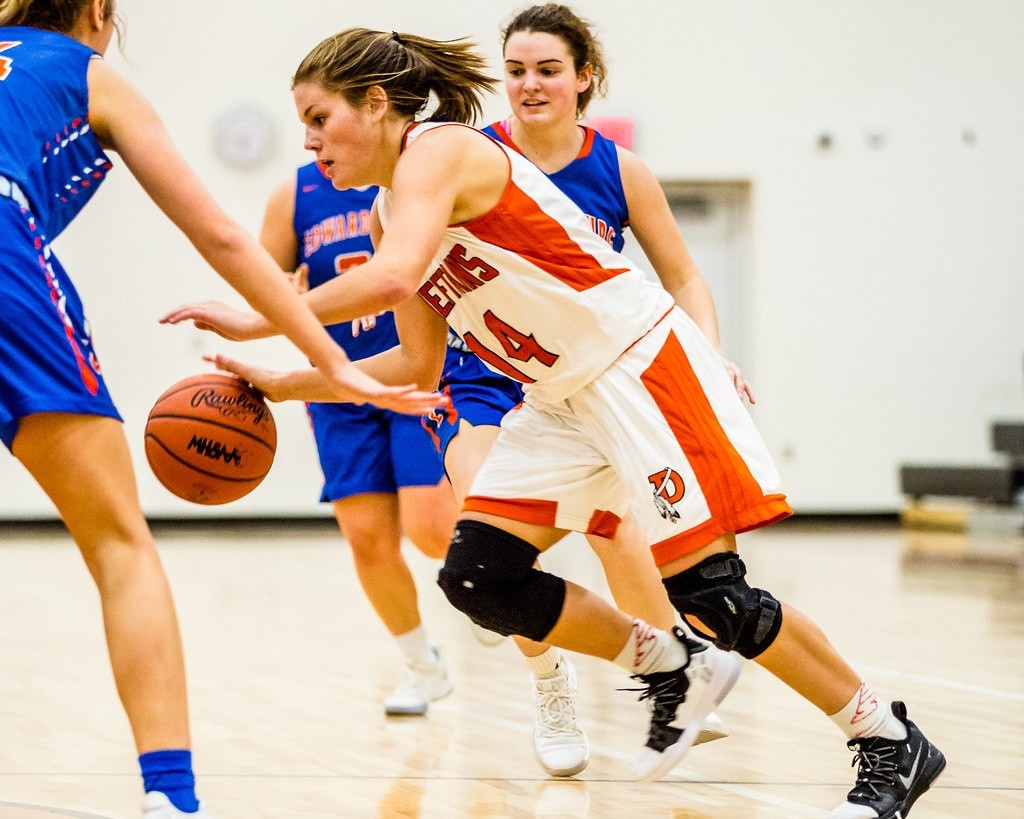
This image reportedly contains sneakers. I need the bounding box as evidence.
[638,626,740,781]
[532,657,589,777]
[834,700,946,819]
[691,716,726,748]
[386,646,453,714]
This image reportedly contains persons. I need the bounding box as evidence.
[0,1,452,817]
[259,160,505,714]
[159,26,947,818]
[420,4,754,779]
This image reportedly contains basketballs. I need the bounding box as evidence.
[143,373,278,506]
